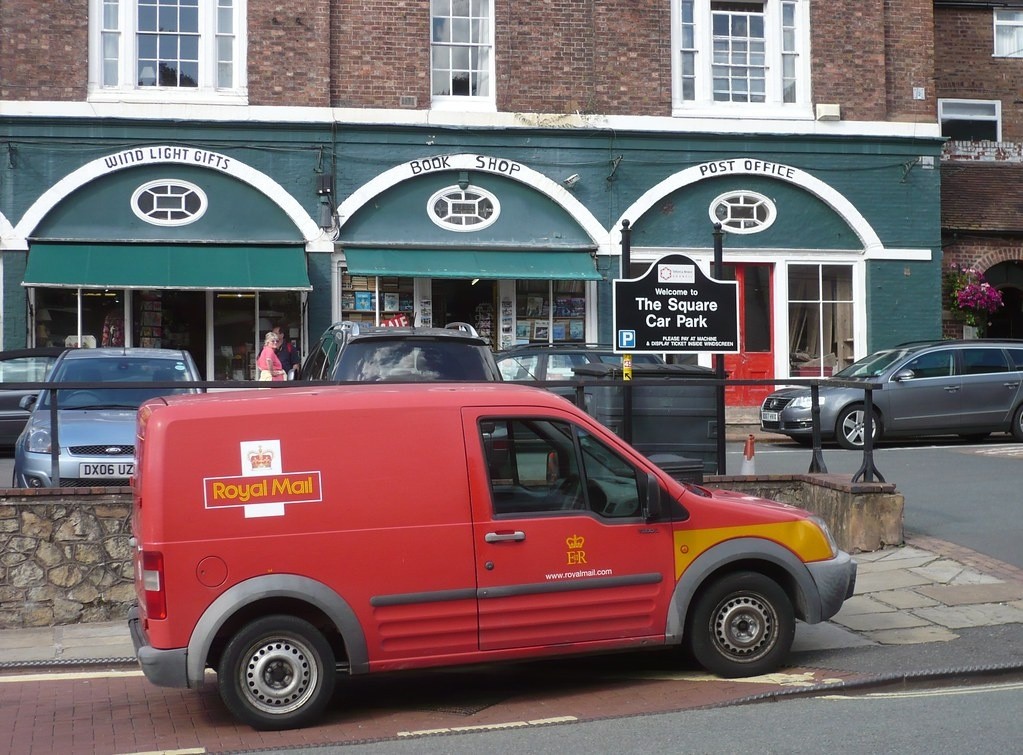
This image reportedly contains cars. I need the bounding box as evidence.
[0,347,77,451]
[12,347,205,488]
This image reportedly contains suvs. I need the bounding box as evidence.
[493,342,668,405]
[759,339,1023,450]
[288,320,504,382]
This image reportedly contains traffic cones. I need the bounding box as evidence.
[741,435,755,475]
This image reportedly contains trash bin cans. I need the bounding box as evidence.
[569,361,733,476]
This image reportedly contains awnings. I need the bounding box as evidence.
[21,243,314,293]
[339,248,603,282]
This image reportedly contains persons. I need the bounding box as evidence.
[258,326,300,381]
[258,332,285,382]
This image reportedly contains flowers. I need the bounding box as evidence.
[944,262,1004,339]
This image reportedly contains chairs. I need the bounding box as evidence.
[80,368,104,394]
[153,370,176,394]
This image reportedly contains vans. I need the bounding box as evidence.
[126,383,857,730]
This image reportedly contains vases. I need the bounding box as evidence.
[963,325,978,339]
